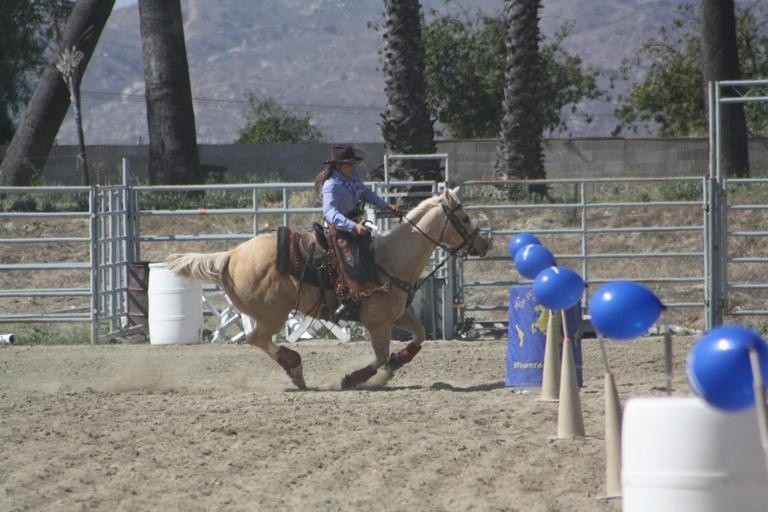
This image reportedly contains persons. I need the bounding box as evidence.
[313,144,403,329]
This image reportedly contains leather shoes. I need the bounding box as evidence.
[334,304,360,321]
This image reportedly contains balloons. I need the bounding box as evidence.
[508,231,541,260]
[513,244,554,279]
[587,281,668,340]
[686,323,768,414]
[533,266,588,310]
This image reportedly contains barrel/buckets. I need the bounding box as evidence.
[621,395,767,511]
[508,284,582,389]
[149,262,204,344]
[126,262,148,337]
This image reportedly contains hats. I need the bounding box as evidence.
[321,144,363,165]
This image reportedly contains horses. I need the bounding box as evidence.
[163,183,490,391]
